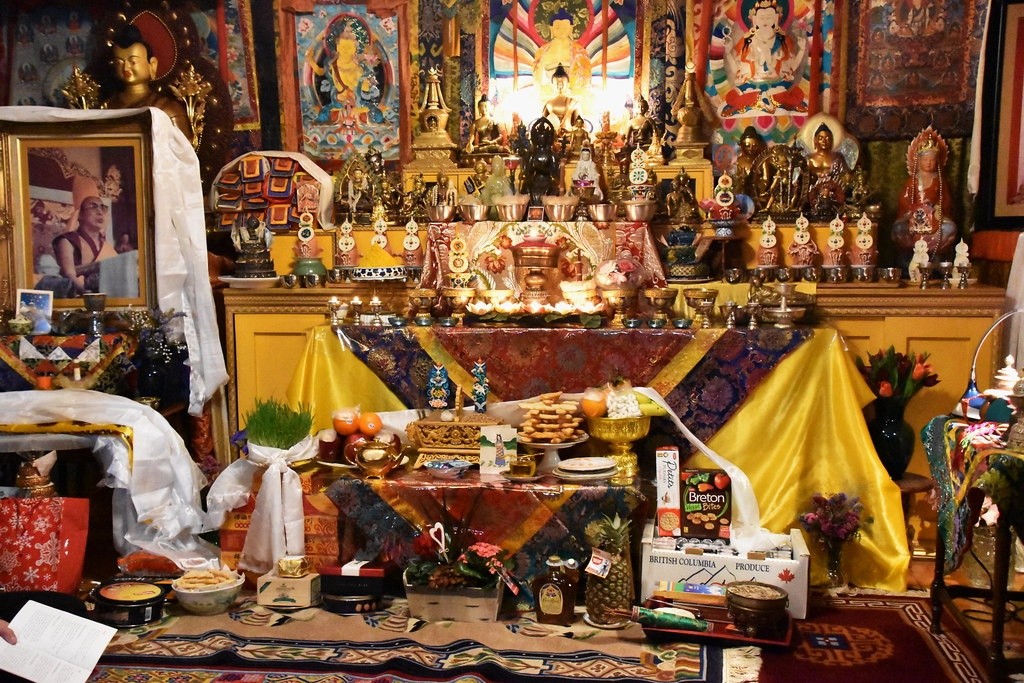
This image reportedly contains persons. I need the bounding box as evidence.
[806,120,852,185]
[470,93,509,152]
[543,63,584,132]
[436,175,451,205]
[379,179,393,210]
[471,161,492,195]
[512,122,531,151]
[624,95,660,147]
[569,116,589,152]
[94,23,195,143]
[737,128,770,192]
[366,148,383,176]
[666,171,698,220]
[351,168,369,196]
[518,128,562,204]
[48,176,120,295]
[889,125,957,254]
[569,146,605,200]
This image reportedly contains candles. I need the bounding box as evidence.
[512,111,520,126]
[370,296,382,305]
[350,296,363,305]
[601,109,611,132]
[464,300,495,315]
[959,260,972,267]
[521,302,551,315]
[546,301,575,315]
[493,301,523,315]
[940,260,952,267]
[574,302,605,316]
[523,228,548,242]
[701,299,713,306]
[918,259,931,268]
[747,300,759,307]
[725,297,738,306]
[328,295,342,306]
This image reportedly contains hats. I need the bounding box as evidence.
[73,173,101,210]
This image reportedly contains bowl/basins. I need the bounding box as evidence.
[83,293,107,311]
[414,316,434,325]
[440,317,459,326]
[425,461,471,478]
[544,204,575,222]
[295,257,327,275]
[646,319,667,327]
[622,318,643,327]
[426,205,457,222]
[495,204,528,222]
[673,319,693,328]
[171,573,245,616]
[586,204,618,222]
[388,317,408,326]
[623,203,657,222]
[9,321,34,334]
[458,204,492,221]
[725,582,790,609]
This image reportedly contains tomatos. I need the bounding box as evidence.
[714,473,730,490]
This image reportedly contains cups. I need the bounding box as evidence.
[510,460,536,477]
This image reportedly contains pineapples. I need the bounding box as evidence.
[586,513,632,624]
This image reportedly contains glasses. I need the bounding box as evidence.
[85,202,110,214]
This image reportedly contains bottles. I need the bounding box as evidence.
[532,556,575,626]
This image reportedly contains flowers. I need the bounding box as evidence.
[798,492,874,550]
[596,260,638,289]
[855,344,941,412]
[404,543,505,588]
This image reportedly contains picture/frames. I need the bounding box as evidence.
[15,289,54,324]
[988,0,1024,222]
[4,123,155,312]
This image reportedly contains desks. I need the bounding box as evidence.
[920,418,1024,683]
[891,472,932,572]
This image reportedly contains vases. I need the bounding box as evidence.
[823,544,845,586]
[683,287,719,328]
[602,290,636,325]
[644,287,678,325]
[406,288,438,317]
[583,413,651,488]
[292,257,329,280]
[869,405,914,481]
[441,286,476,321]
[402,567,507,623]
[479,288,515,305]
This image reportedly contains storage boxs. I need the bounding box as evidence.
[641,511,810,620]
[279,556,311,578]
[257,572,322,607]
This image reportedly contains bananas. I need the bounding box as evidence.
[634,394,667,416]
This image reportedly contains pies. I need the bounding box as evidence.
[175,569,230,591]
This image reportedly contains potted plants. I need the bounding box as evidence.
[241,395,316,463]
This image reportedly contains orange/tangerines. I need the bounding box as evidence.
[332,410,382,435]
[579,389,608,418]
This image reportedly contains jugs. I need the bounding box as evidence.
[344,437,412,480]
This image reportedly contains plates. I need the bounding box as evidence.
[583,613,633,628]
[499,470,546,482]
[218,276,279,288]
[552,456,618,486]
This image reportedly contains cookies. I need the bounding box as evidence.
[516,391,585,444]
[687,513,729,530]
[659,512,679,531]
